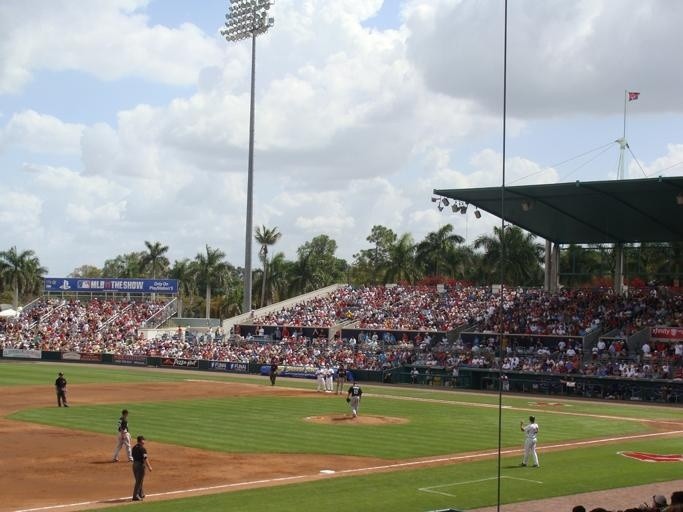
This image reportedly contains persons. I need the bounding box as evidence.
[1,294,167,353]
[571,490,682,512]
[519,414,540,469]
[110,409,134,464]
[248,279,683,327]
[159,325,681,403]
[54,371,68,408]
[130,434,153,502]
[345,381,363,418]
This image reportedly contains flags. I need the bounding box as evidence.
[627,91,641,102]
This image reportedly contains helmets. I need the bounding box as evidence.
[529,416,535,421]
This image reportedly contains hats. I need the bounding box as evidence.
[59,372,64,375]
[137,436,145,441]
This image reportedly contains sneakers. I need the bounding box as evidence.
[133,494,145,501]
[522,464,539,468]
[113,458,133,462]
[59,405,68,407]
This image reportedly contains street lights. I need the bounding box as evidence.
[220,0,275,315]
